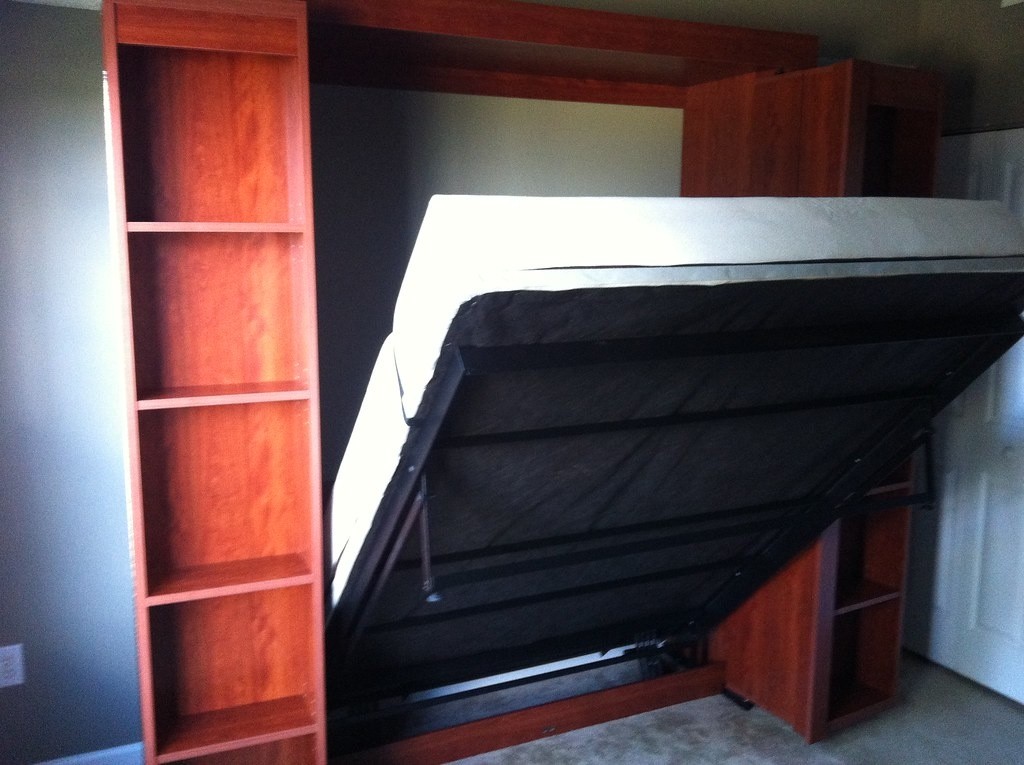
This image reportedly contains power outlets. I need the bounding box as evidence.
[0,643,25,687]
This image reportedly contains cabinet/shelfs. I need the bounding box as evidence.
[101,0,950,765]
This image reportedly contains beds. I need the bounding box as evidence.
[327,193,1024,729]
